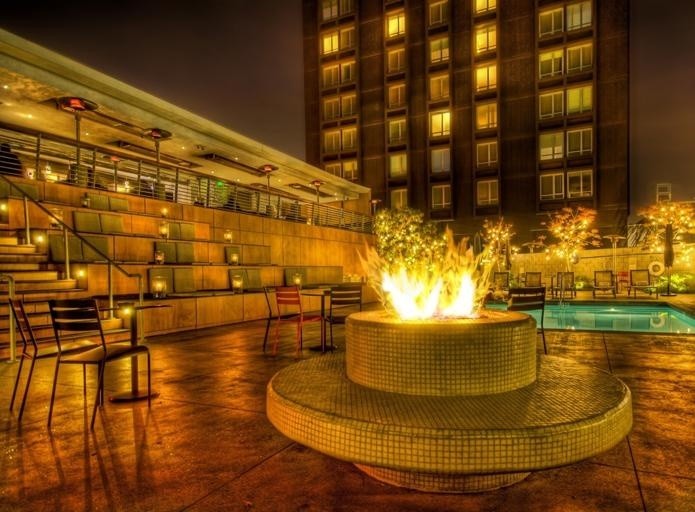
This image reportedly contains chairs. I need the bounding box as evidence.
[508,288,549,353]
[525,272,542,288]
[558,271,576,300]
[47,301,153,428]
[626,270,658,299]
[594,270,614,300]
[262,283,362,360]
[494,271,509,297]
[8,298,106,420]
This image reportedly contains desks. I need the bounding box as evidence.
[96,305,173,403]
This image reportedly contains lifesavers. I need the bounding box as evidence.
[649,261,665,276]
